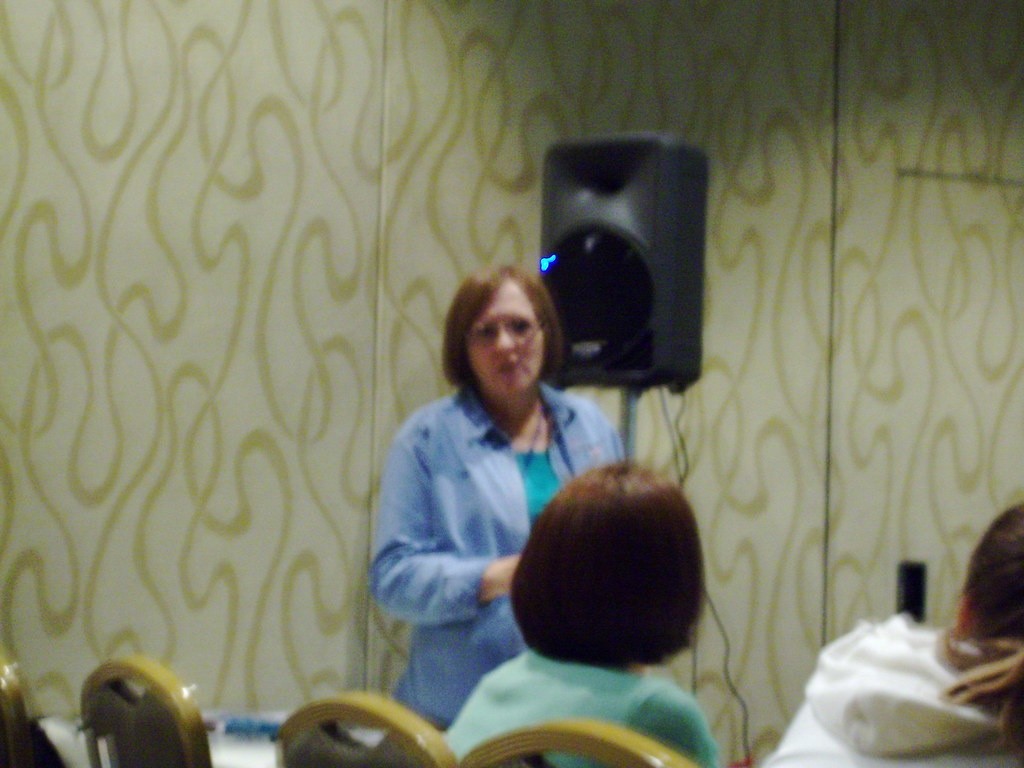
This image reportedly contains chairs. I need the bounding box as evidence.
[275,688,460,768]
[81,655,212,768]
[0,658,36,768]
[460,718,698,768]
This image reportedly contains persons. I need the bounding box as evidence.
[764,503,1024,768]
[443,461,726,768]
[367,267,629,727]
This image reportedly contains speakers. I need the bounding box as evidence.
[540,134,709,396]
